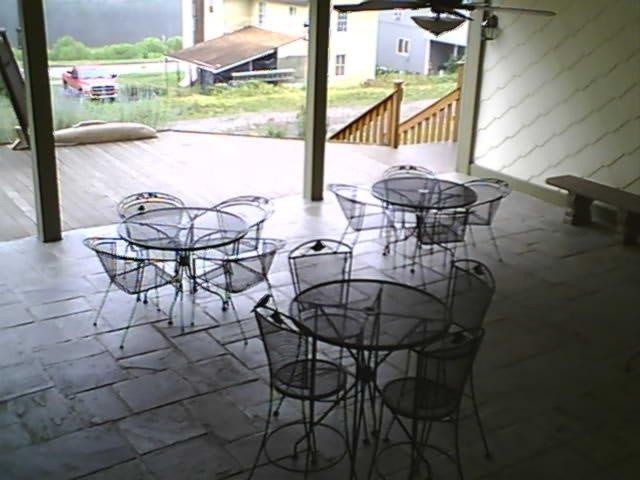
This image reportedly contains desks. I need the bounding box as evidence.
[370,177,478,273]
[289,279,453,480]
[116,206,249,325]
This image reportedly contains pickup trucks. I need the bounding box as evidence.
[62,64,121,103]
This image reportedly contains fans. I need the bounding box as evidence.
[332,1,555,21]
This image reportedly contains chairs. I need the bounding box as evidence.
[442,177,512,262]
[181,237,286,347]
[383,209,476,275]
[381,257,495,462]
[192,195,274,306]
[327,184,397,257]
[274,238,362,415]
[365,327,492,480]
[83,235,194,352]
[381,165,440,253]
[116,192,192,304]
[248,303,360,480]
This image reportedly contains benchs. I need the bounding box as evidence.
[545,175,640,245]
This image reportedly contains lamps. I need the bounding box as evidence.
[481,16,498,41]
[412,10,465,35]
